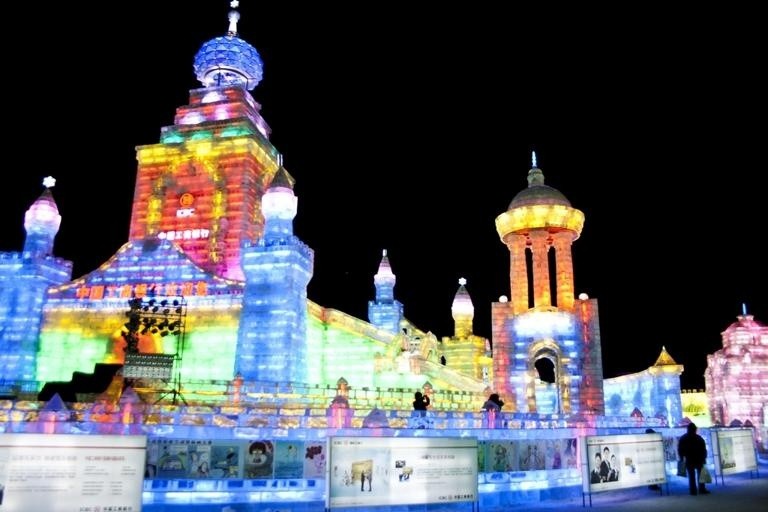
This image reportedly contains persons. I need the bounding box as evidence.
[678,423,710,495]
[413,392,430,409]
[361,469,372,492]
[482,394,504,412]
[590,447,618,484]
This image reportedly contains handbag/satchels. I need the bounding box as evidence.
[677,461,687,477]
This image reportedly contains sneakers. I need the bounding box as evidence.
[689,488,710,496]
[648,486,660,491]
